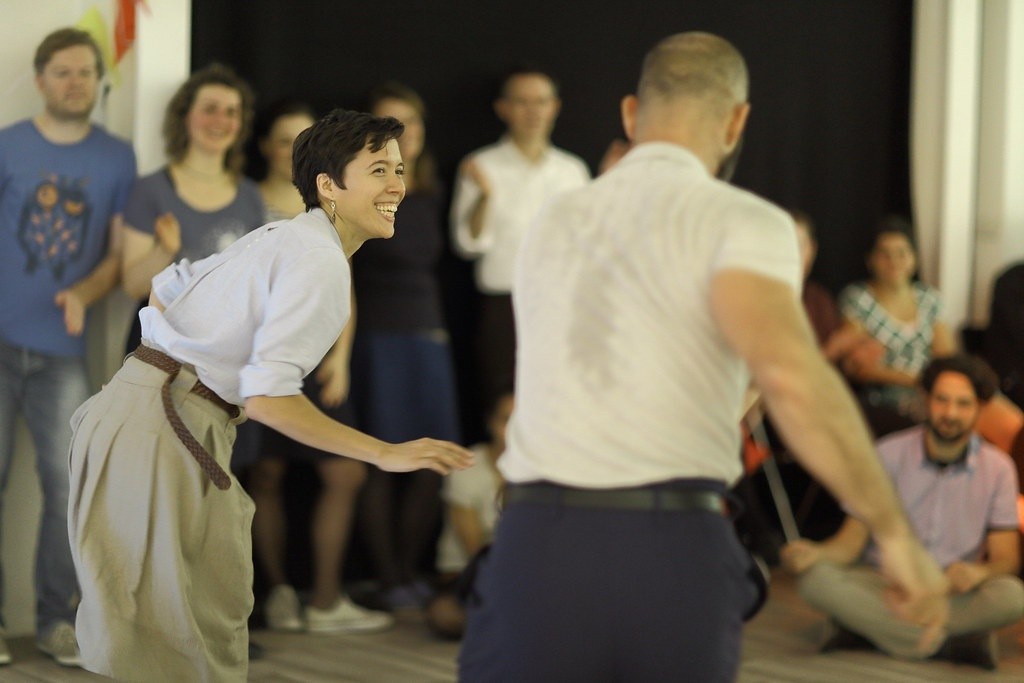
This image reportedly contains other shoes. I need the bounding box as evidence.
[303,599,394,633]
[422,595,462,635]
[0,631,12,664]
[817,624,874,654]
[263,582,303,633]
[32,619,79,668]
[948,633,997,670]
[397,604,423,621]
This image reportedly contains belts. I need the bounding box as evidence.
[123,342,242,492]
[502,480,733,517]
[411,325,450,347]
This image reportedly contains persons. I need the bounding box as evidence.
[785,216,1022,542]
[455,33,950,683]
[252,73,624,642]
[123,65,264,354]
[0,28,138,664]
[66,110,474,683]
[779,354,1024,671]
